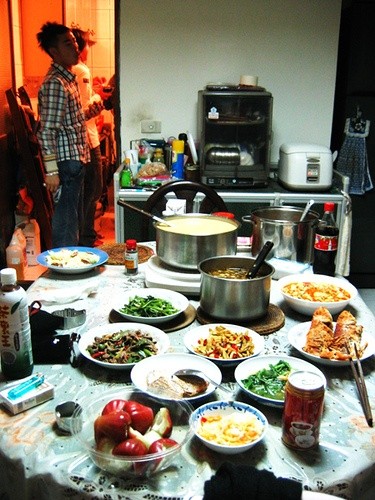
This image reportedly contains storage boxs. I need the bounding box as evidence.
[0,374,56,415]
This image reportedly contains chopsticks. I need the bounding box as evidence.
[343,339,373,427]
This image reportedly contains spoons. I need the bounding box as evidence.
[176,369,235,396]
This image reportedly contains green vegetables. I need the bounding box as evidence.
[89,330,155,364]
[120,295,178,317]
[242,360,296,400]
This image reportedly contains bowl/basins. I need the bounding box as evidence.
[110,287,189,324]
[234,354,327,410]
[71,385,197,479]
[278,275,358,317]
[78,320,265,370]
[36,246,110,274]
[189,400,269,455]
[129,352,223,403]
[287,317,375,367]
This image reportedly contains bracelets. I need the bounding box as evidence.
[45,171,59,177]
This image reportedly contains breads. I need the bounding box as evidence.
[150,373,206,398]
[139,163,165,177]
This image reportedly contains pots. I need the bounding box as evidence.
[152,210,242,272]
[196,256,276,324]
[242,206,321,267]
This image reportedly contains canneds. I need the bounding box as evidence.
[280,369,325,451]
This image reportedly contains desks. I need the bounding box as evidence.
[113,163,350,253]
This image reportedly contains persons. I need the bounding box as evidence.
[70,28,103,247]
[36,21,104,249]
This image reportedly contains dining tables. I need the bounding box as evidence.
[0,241,375,500]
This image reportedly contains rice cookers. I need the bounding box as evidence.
[277,141,338,191]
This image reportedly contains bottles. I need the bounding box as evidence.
[125,239,138,273]
[119,144,163,189]
[313,202,339,276]
[0,268,34,379]
